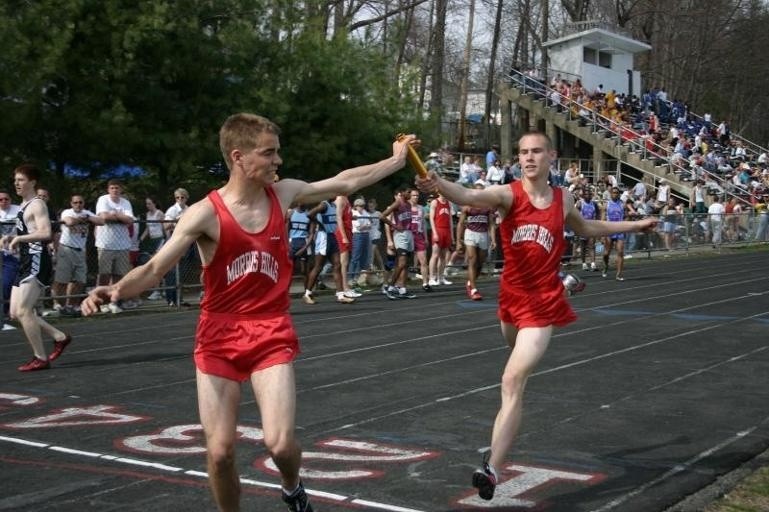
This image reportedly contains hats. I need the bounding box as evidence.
[657,178,667,184]
[475,178,486,185]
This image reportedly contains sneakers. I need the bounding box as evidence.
[583,262,624,281]
[559,260,571,266]
[49,333,71,360]
[17,356,50,371]
[2,324,17,330]
[471,462,496,500]
[42,291,189,317]
[282,479,313,512]
[303,263,482,304]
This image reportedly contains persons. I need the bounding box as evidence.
[0,165,72,371]
[274,174,432,305]
[80,113,421,512]
[414,131,660,500]
[1,178,204,324]
[422,63,769,301]
